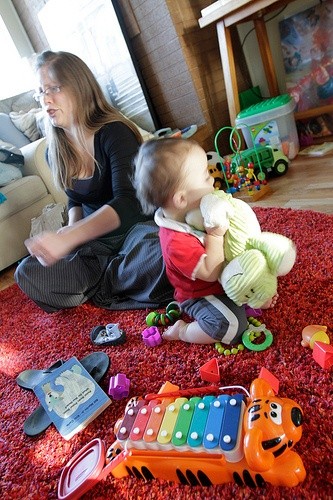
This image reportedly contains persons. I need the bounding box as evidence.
[12,49,176,312]
[131,137,279,344]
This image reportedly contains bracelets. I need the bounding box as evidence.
[215,316,261,355]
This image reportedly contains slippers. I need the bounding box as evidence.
[23,351,111,438]
[17,359,67,392]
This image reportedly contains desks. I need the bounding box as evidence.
[199,0,333,150]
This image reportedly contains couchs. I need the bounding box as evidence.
[0,90,71,272]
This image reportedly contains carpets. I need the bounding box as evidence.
[0,205,333,500]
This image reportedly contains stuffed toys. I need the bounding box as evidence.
[186,190,297,309]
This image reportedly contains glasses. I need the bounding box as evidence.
[35,85,62,102]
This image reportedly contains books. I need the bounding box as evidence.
[32,355,112,442]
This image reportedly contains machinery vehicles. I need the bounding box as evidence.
[232,146,290,182]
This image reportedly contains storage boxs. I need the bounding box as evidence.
[235,95,301,160]
